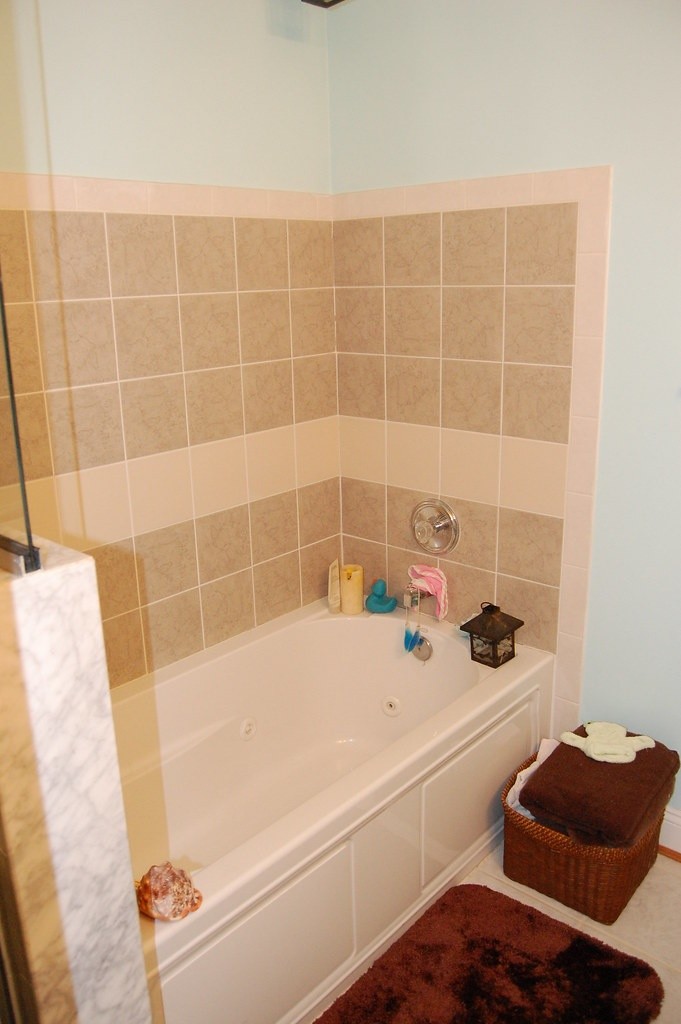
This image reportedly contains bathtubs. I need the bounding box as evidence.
[110,593,553,1024]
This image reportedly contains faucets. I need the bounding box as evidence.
[403,587,433,607]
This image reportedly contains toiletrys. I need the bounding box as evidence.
[339,564,364,615]
[328,558,341,614]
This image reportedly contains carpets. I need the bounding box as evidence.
[312,884,665,1024]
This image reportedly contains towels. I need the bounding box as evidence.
[407,564,449,619]
[505,721,681,850]
